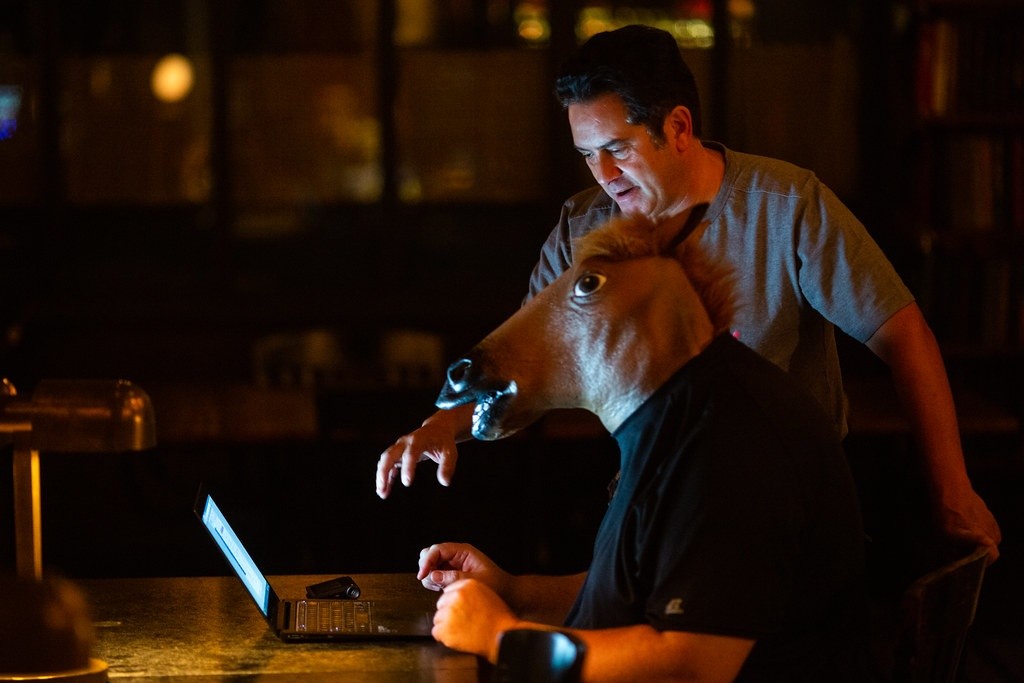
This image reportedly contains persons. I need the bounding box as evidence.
[377,23,1002,568]
[416,204,876,683]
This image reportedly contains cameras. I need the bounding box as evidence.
[306,576,361,599]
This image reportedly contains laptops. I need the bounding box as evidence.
[193,484,439,642]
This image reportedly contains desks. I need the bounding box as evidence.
[44,574,527,683]
